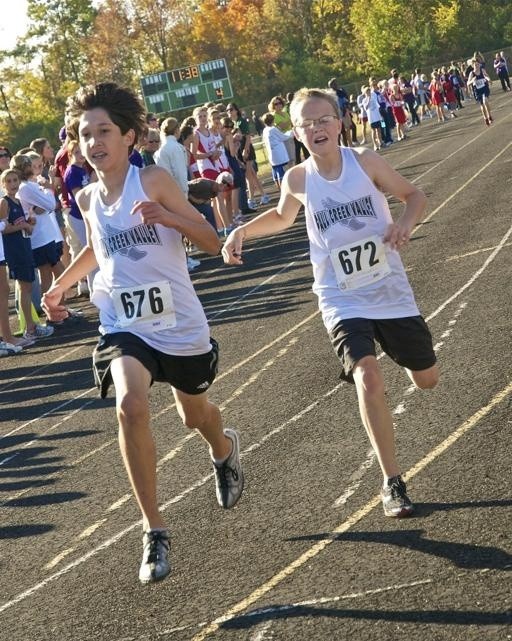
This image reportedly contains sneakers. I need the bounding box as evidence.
[217,207,258,237]
[138,528,171,584]
[0,342,23,358]
[45,280,89,327]
[350,132,410,151]
[405,94,474,128]
[247,199,259,209]
[381,474,415,518]
[23,323,54,340]
[260,195,271,204]
[209,428,245,508]
[483,115,494,126]
[187,256,201,271]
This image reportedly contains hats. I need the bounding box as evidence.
[59,125,67,141]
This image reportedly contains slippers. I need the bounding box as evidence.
[11,337,34,347]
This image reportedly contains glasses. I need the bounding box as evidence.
[294,115,339,130]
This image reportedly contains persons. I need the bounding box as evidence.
[1,126,101,357]
[220,87,439,518]
[39,83,244,586]
[330,52,493,148]
[492,53,512,93]
[138,91,310,277]
[499,50,510,76]
